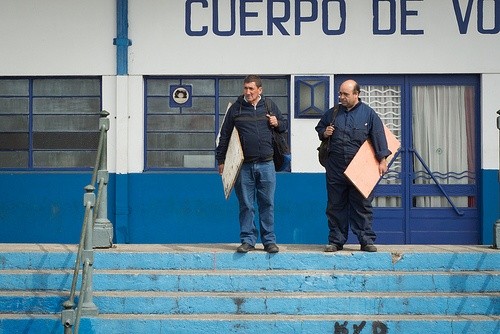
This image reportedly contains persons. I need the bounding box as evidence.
[314,79,392,252]
[214,74,290,253]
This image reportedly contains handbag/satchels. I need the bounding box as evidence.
[316,105,341,167]
[264,96,291,172]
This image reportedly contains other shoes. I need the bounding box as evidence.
[264,242,279,253]
[360,243,377,252]
[237,242,255,252]
[324,243,342,252]
